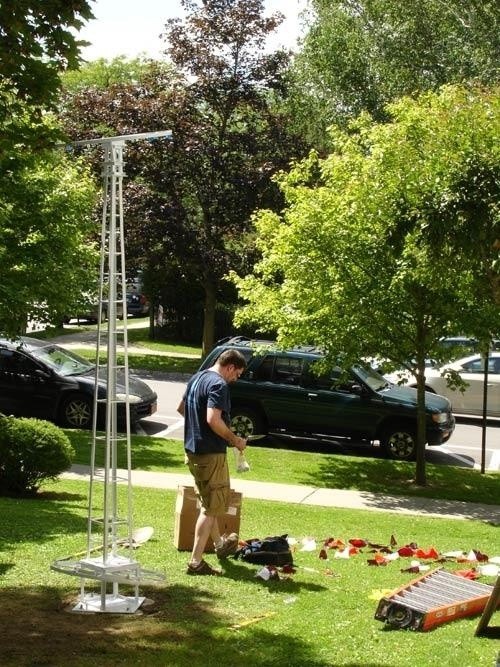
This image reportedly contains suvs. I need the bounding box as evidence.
[198,336,455,460]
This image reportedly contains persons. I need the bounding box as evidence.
[177,347,247,577]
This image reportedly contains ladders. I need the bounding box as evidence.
[374,566,500,632]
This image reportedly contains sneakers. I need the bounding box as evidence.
[186,559,223,576]
[216,534,239,559]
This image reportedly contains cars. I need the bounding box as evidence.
[384,352,500,417]
[0,336,157,429]
[61,283,123,323]
[117,288,149,320]
